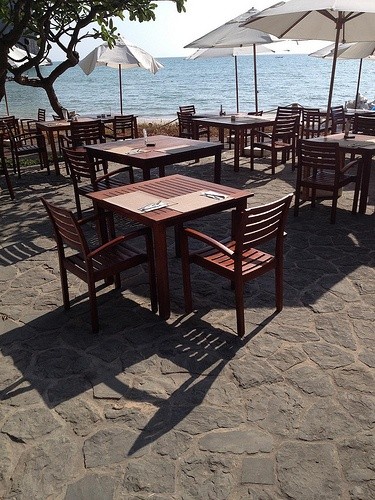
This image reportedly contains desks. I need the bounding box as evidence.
[303,132,375,216]
[97,116,138,139]
[194,114,276,172]
[84,135,223,185]
[84,174,255,320]
[36,116,105,175]
[310,111,345,135]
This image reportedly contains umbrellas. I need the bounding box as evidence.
[183,41,308,114]
[3,45,53,116]
[308,41,375,109]
[238,0,375,135]
[76,33,165,136]
[182,6,314,117]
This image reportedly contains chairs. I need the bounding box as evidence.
[39,195,159,320]
[177,105,331,174]
[62,148,134,222]
[294,139,361,224]
[181,193,294,338]
[330,106,346,133]
[351,113,375,166]
[0,108,134,201]
